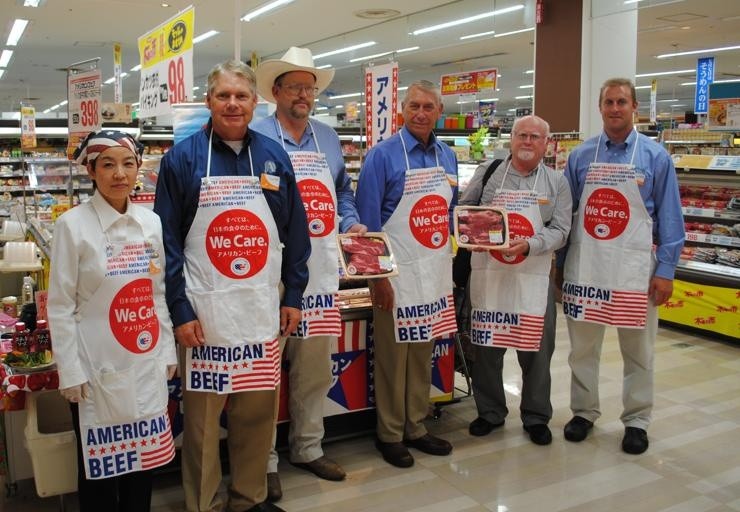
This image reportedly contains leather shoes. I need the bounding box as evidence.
[564,416,593,442]
[289,455,345,481]
[469,417,505,435]
[228,472,286,512]
[523,423,552,445]
[375,433,453,468]
[622,427,648,454]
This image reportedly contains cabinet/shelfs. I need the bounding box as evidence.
[633,120,740,279]
[0,129,173,232]
[334,134,498,196]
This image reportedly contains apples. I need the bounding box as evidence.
[45,371,59,390]
[27,374,47,392]
[8,375,25,388]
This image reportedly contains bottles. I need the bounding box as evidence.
[1,272,52,364]
[436,113,479,128]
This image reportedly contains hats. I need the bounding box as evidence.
[254,46,335,104]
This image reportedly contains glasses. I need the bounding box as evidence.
[278,82,319,98]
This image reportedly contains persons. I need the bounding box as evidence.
[249,47,366,481]
[556,78,686,454]
[354,80,458,470]
[46,129,177,512]
[153,60,312,512]
[459,115,573,445]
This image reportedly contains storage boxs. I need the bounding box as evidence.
[22,387,80,499]
